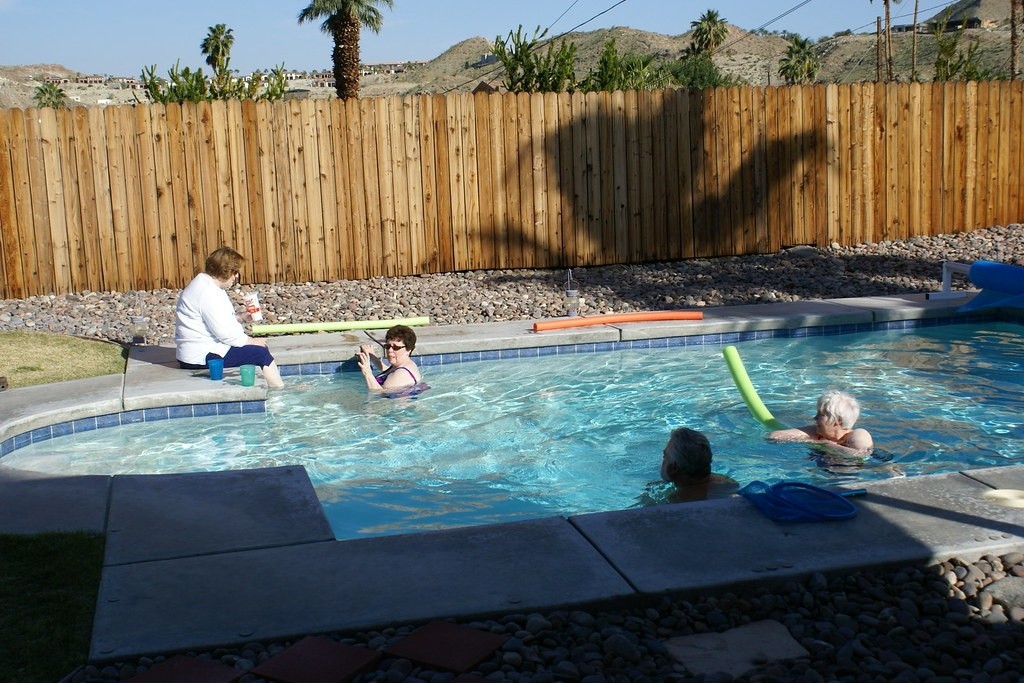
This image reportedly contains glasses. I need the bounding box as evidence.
[383,343,407,351]
[229,268,239,277]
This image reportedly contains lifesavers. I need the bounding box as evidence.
[532,309,704,333]
[250,316,431,336]
[721,344,878,463]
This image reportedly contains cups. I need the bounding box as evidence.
[244,291,262,321]
[208,359,224,380]
[240,364,255,386]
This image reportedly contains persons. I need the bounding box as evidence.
[769,389,873,450]
[355,325,421,391]
[175,246,284,387]
[661,427,739,500]
